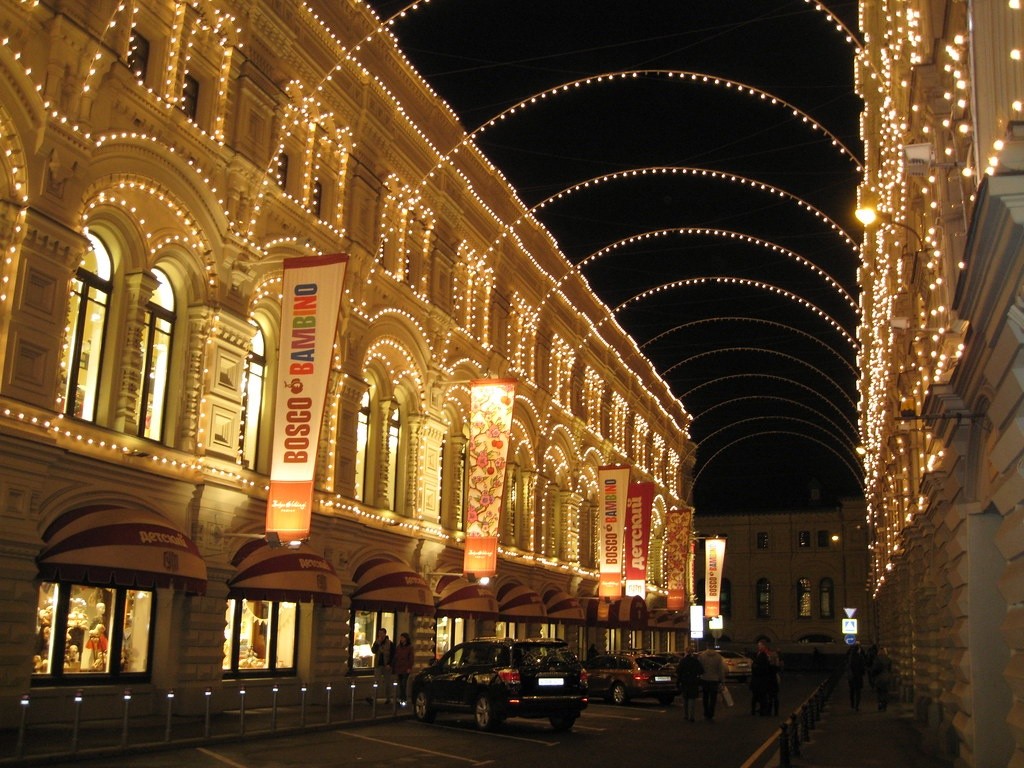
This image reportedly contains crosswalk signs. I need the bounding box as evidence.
[842,619,857,635]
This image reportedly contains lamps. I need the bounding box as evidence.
[904,142,974,178]
[425,564,498,587]
[216,522,309,551]
[854,208,925,253]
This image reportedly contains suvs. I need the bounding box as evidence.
[408,636,588,729]
[586,653,679,705]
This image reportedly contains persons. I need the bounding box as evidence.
[366,628,395,703]
[866,643,892,710]
[676,646,704,722]
[393,633,415,703]
[588,643,599,658]
[749,639,786,717]
[846,640,868,712]
[812,647,820,665]
[85,603,108,669]
[697,643,724,720]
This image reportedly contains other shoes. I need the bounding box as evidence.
[365,697,374,704]
[751,710,755,716]
[690,718,695,722]
[878,702,886,710]
[851,703,859,711]
[683,716,688,720]
[397,700,408,707]
[384,700,391,705]
[703,715,715,720]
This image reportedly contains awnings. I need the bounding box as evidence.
[579,595,648,630]
[33,501,207,598]
[648,609,689,631]
[350,553,586,623]
[226,538,342,606]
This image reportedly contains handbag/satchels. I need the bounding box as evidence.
[722,686,734,707]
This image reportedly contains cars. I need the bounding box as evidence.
[695,650,754,682]
[655,653,682,667]
